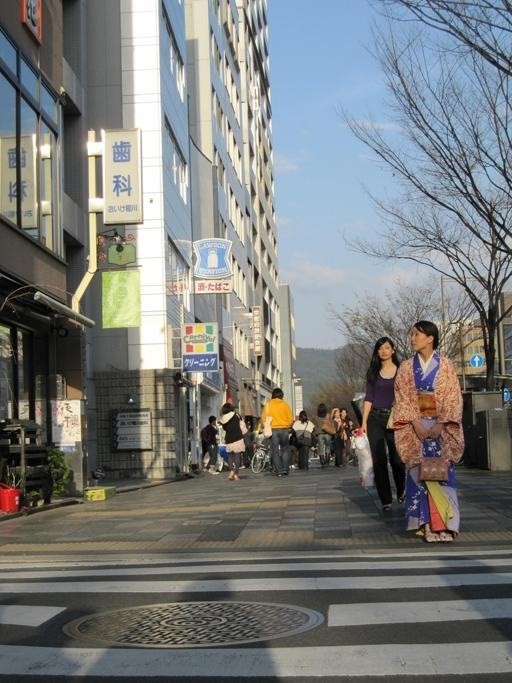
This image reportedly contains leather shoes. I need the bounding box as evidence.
[277,472,288,477]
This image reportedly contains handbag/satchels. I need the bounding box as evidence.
[263,416,272,438]
[418,456,449,482]
[321,420,336,435]
[236,413,248,435]
[293,432,304,449]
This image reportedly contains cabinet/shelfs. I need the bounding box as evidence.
[5,423,53,505]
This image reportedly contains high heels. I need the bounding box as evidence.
[383,503,393,512]
[397,490,406,504]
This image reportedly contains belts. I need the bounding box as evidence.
[372,408,390,414]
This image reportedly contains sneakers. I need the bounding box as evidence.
[229,470,239,481]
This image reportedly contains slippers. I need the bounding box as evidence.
[424,529,454,543]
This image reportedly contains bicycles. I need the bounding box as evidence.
[251,443,271,473]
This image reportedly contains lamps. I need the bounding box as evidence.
[98,229,124,253]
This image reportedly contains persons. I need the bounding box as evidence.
[361,337,406,512]
[387,321,465,543]
[201,388,358,481]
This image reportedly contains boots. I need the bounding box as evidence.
[320,452,330,468]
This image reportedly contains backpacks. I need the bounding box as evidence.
[201,425,212,447]
